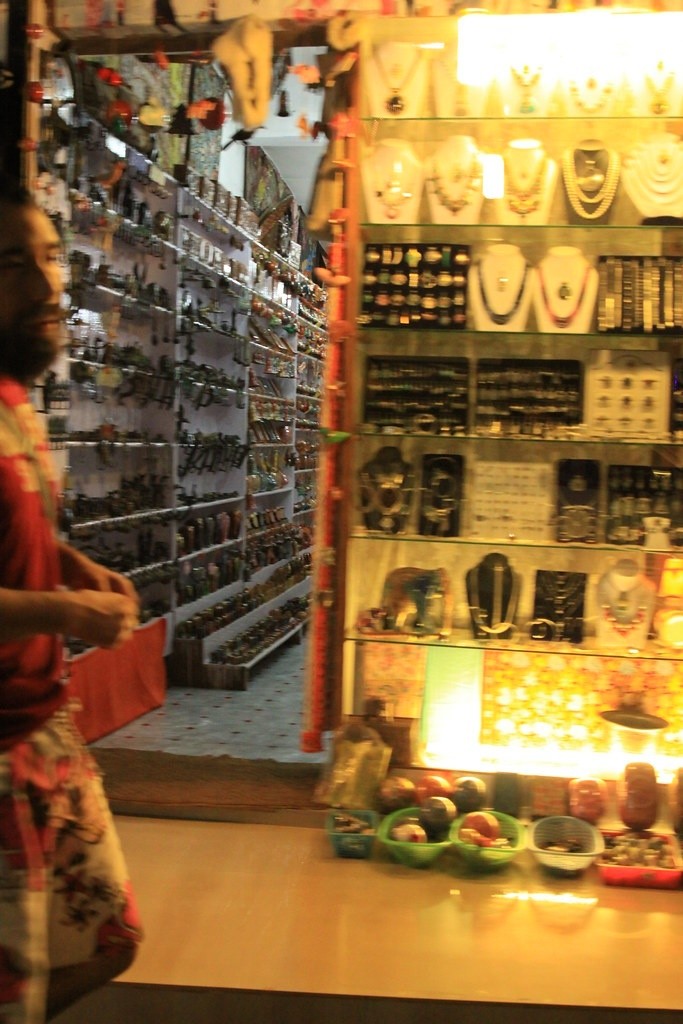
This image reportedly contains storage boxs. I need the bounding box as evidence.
[169,164,304,445]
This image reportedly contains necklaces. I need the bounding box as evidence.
[351,40,683,660]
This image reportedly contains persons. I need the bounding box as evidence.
[0,188,145,1024]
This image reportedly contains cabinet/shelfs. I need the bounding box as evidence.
[335,112,683,661]
[44,105,326,656]
[175,576,311,687]
